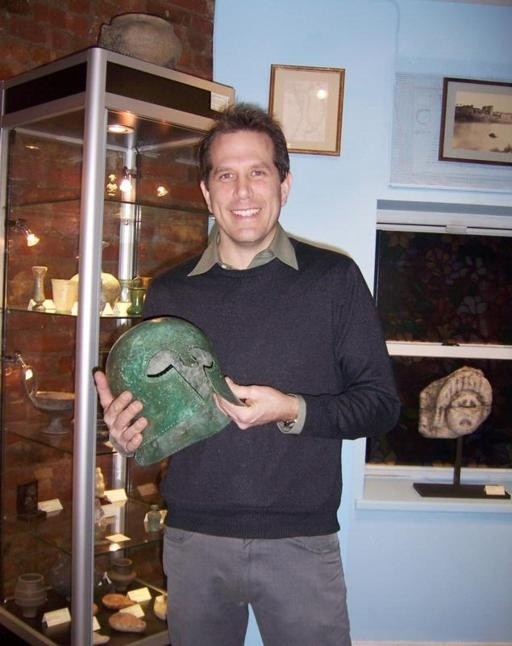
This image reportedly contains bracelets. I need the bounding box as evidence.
[285,419,296,428]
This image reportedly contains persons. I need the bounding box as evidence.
[92,103,402,646]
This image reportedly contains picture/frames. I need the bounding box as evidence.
[438,77,512,166]
[266,63,346,157]
